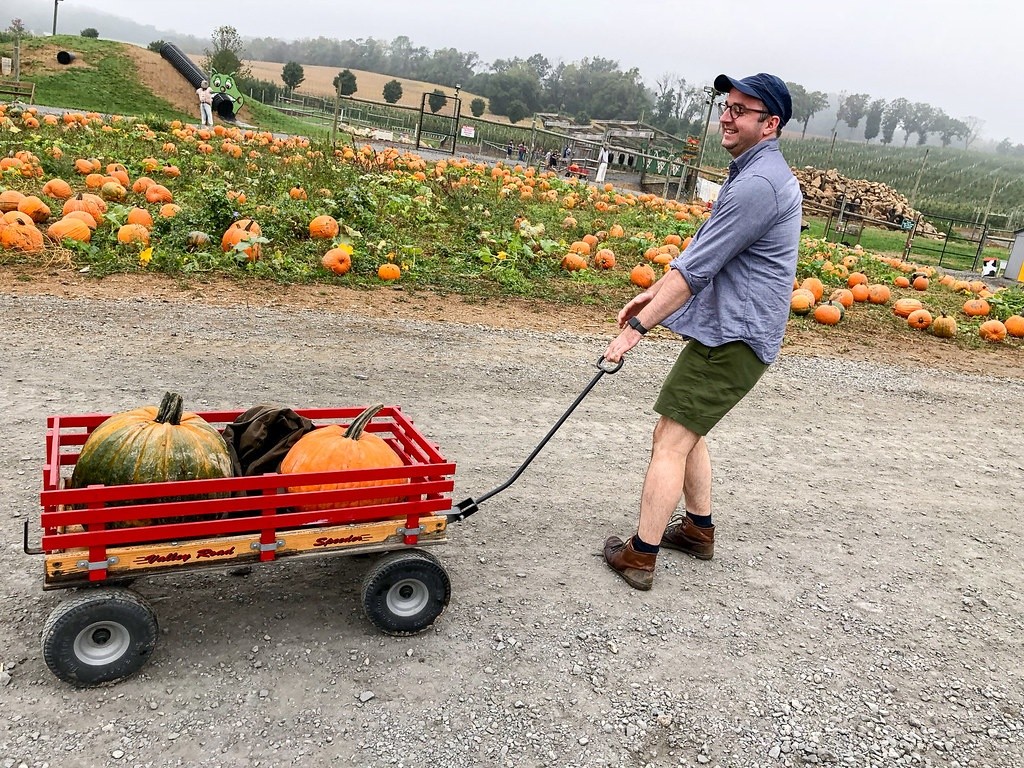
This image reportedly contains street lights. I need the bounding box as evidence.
[53,0,64,35]
[449,83,462,140]
[688,85,726,202]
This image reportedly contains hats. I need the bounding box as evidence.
[714,72,792,130]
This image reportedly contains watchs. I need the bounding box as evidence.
[628,316,649,335]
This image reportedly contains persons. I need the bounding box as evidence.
[602,70,805,591]
[195,80,215,126]
[507,139,578,170]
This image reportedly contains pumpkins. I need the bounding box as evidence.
[280,403,408,513]
[70,390,234,547]
[0,107,711,288]
[787,239,1024,342]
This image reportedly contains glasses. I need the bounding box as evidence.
[718,102,773,119]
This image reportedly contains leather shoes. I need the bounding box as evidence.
[659,514,716,560]
[604,535,658,591]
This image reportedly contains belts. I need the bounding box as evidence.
[201,102,210,105]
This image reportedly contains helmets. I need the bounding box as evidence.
[603,142,609,150]
[201,79,209,88]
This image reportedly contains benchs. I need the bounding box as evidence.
[0,80,35,106]
